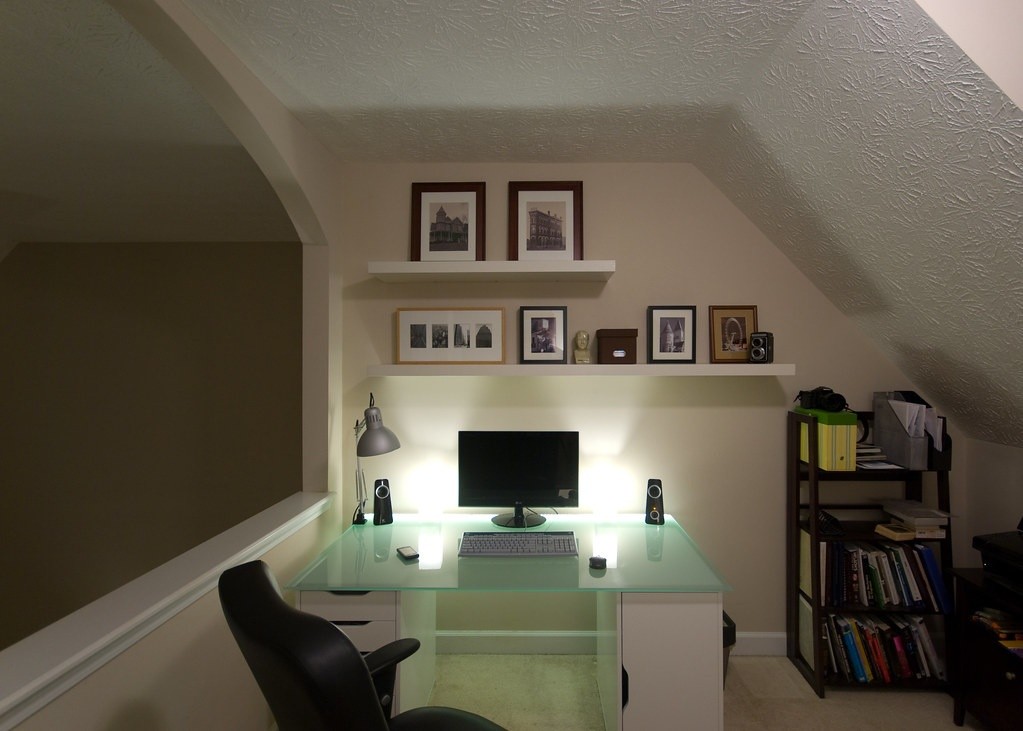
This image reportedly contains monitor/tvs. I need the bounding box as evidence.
[458,431,579,528]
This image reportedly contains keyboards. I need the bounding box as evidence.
[458,531,578,556]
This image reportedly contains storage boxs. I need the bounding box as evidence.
[793,403,857,472]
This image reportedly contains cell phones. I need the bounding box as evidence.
[397,546,419,559]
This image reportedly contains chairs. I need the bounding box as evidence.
[218,560,506,731]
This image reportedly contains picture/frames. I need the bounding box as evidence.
[508,180,583,261]
[410,181,485,261]
[709,304,758,363]
[519,305,568,364]
[396,307,506,365]
[647,305,696,363]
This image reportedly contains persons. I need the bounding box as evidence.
[573,332,591,365]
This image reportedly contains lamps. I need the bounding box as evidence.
[352,392,401,524]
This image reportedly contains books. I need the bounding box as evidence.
[819,527,948,685]
[856,444,888,462]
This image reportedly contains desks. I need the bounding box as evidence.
[282,515,727,731]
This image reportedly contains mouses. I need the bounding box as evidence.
[589,557,606,570]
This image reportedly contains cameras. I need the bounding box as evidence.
[799,386,846,413]
[749,332,774,364]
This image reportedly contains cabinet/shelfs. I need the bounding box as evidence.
[949,531,1023,731]
[785,412,957,698]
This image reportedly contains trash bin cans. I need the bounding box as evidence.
[722,611,736,689]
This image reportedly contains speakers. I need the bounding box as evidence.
[645,479,665,525]
[373,479,393,525]
